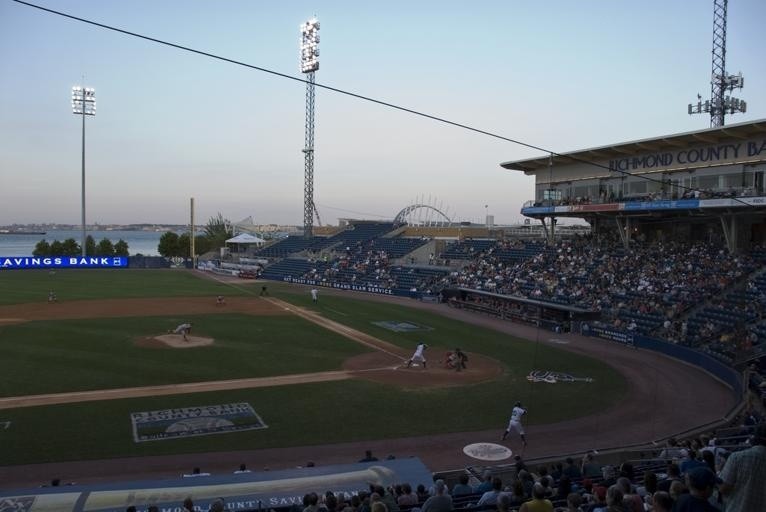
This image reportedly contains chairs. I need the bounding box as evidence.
[254,384,763,512]
[253,221,763,384]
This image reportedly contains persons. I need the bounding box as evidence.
[47,180,766,512]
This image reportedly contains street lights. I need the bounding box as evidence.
[71,84,96,257]
